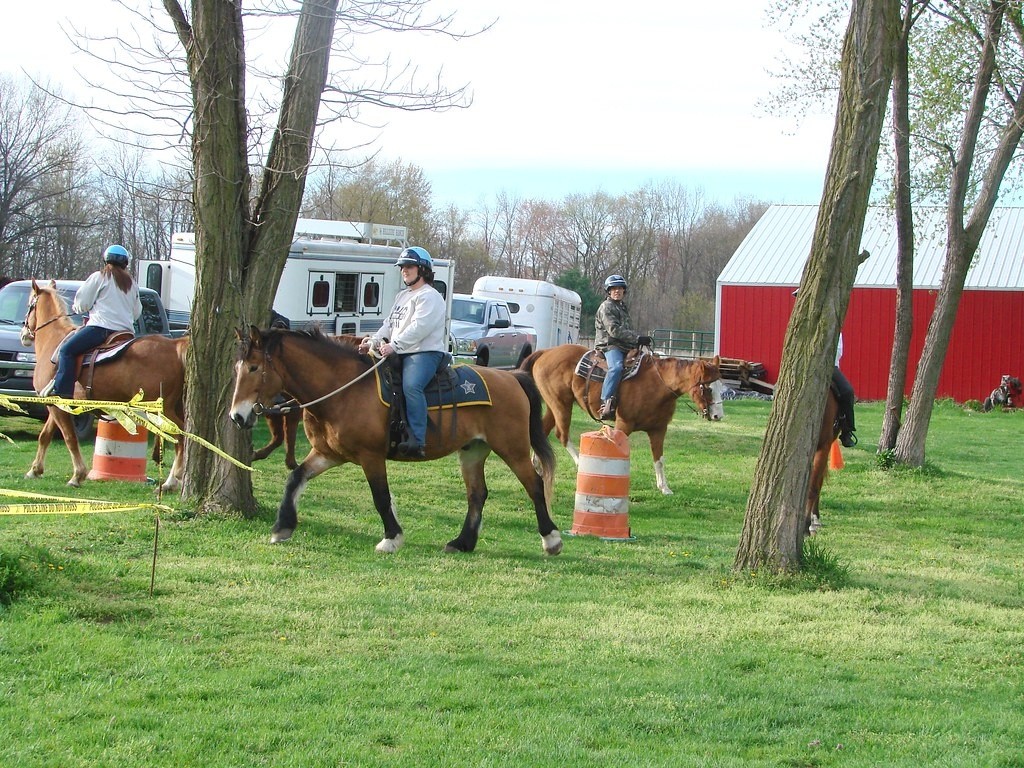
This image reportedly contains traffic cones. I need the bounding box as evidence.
[829,438,845,470]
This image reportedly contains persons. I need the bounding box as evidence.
[792,289,856,448]
[48,245,143,399]
[594,274,652,417]
[357,246,446,457]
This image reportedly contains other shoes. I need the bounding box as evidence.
[38,392,74,406]
[399,442,426,458]
[840,433,855,447]
[597,403,616,420]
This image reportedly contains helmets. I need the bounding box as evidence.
[104,244,129,269]
[791,288,799,298]
[394,246,433,272]
[604,275,627,294]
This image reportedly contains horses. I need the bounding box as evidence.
[520,343,727,496]
[19,275,300,495]
[229,325,567,558]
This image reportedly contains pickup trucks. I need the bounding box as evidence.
[446,293,538,371]
[0,279,191,444]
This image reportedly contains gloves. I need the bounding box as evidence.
[637,336,651,347]
